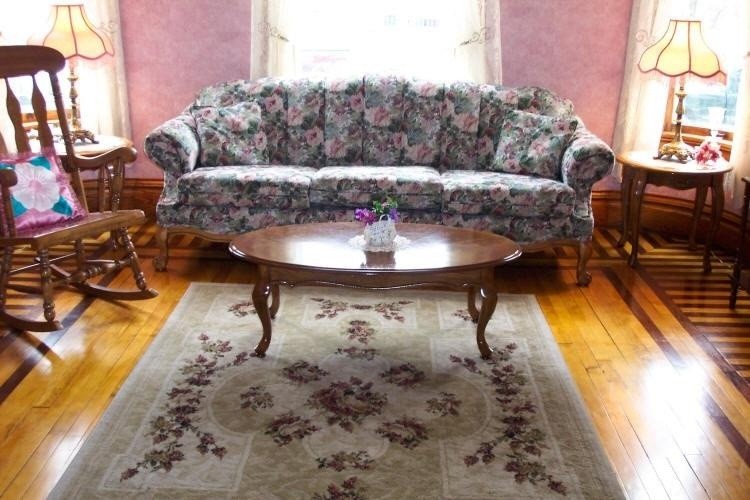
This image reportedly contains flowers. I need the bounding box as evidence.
[356,198,399,224]
[695,143,719,164]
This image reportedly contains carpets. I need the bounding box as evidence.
[43,283,629,500]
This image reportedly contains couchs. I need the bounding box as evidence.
[144,77,614,287]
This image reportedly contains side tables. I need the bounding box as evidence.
[29,135,136,251]
[617,152,732,274]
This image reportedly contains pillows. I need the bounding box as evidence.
[0,149,85,235]
[191,102,270,165]
[489,107,577,178]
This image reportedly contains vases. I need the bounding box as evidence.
[364,222,397,247]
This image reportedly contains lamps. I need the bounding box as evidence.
[638,20,720,165]
[27,4,115,145]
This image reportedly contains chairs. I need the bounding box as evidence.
[0,45,157,330]
